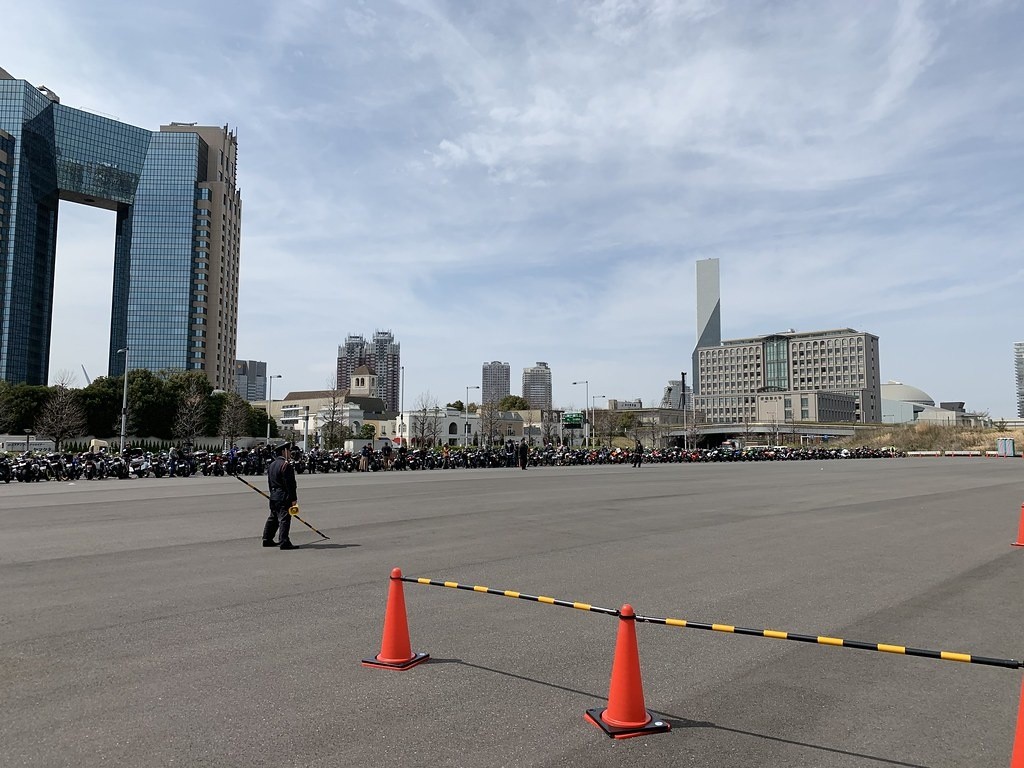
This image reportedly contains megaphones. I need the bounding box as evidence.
[288,503,300,516]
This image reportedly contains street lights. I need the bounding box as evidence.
[573,380,589,447]
[267,374,283,447]
[116,347,130,455]
[592,396,606,449]
[466,386,480,448]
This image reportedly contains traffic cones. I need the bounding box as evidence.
[584,603,670,739]
[1010,502,1024,546]
[1008,674,1024,768]
[361,567,430,671]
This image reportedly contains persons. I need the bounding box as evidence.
[890,444,896,458]
[442,443,451,469]
[556,442,570,466]
[382,442,392,471]
[169,444,179,478]
[262,441,300,550]
[544,442,555,466]
[632,440,644,468]
[227,443,241,476]
[250,442,269,475]
[122,444,132,479]
[359,442,373,472]
[308,446,320,474]
[519,437,528,470]
[71,451,84,480]
[399,442,408,471]
[505,439,514,468]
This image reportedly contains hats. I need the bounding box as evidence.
[273,442,291,452]
[675,446,678,448]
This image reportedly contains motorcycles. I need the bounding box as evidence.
[0,442,909,484]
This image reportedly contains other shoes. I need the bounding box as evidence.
[633,464,636,467]
[280,544,300,550]
[522,467,527,470]
[638,465,640,467]
[263,539,280,547]
